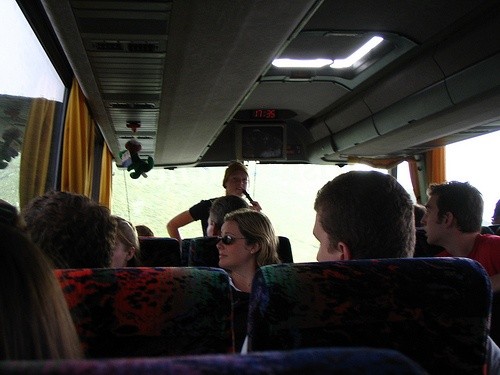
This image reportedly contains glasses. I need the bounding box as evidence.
[215,235,247,245]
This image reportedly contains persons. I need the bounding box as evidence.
[206,194,252,238]
[166,162,262,241]
[216,208,282,354]
[0,190,155,360]
[312,170,500,375]
[420,180,500,292]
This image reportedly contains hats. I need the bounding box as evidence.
[223,163,248,186]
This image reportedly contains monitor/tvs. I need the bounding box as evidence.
[234,121,287,162]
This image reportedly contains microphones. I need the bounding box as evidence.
[242,189,253,201]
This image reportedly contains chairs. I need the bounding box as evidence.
[0,226,495,375]
